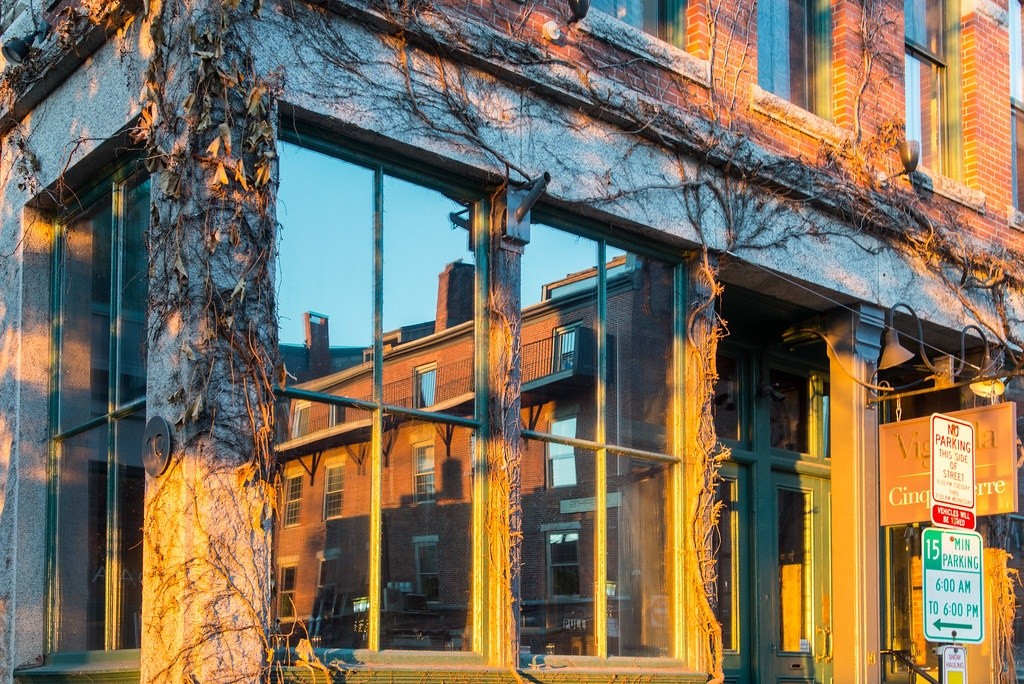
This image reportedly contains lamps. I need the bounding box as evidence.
[542,0,589,40]
[2,21,52,67]
[877,303,938,376]
[873,140,920,187]
[759,380,786,405]
[954,325,1005,397]
